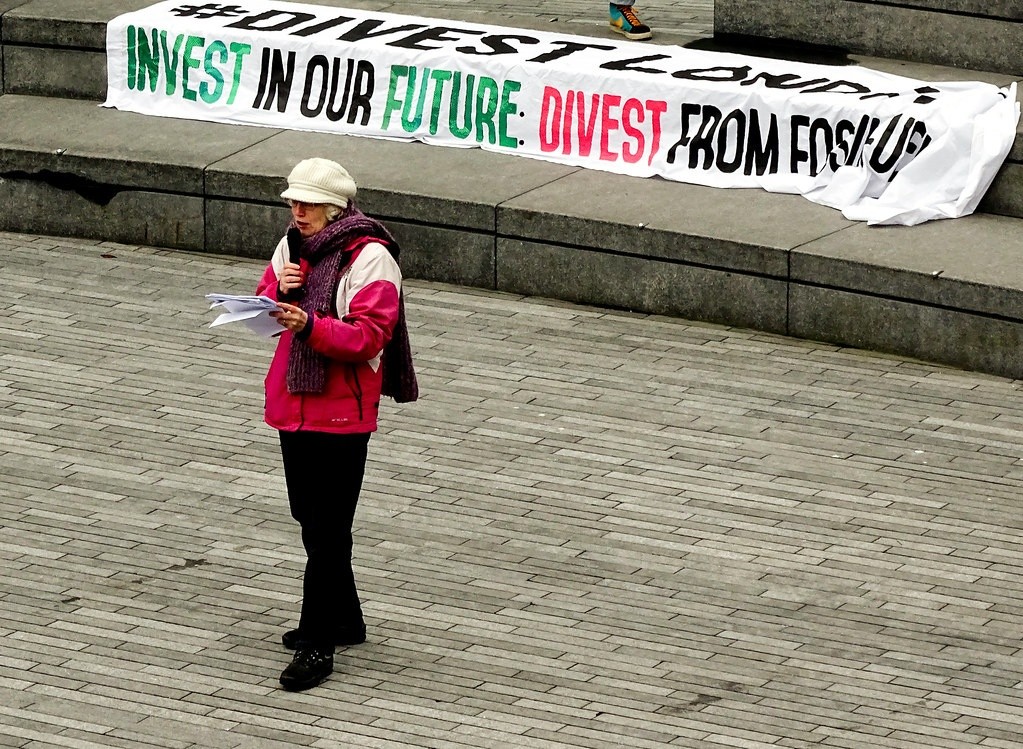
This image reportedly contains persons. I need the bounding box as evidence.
[254,157,419,691]
[610,0,652,39]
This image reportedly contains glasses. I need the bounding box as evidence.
[286,199,325,211]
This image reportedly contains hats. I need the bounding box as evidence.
[280,158,356,209]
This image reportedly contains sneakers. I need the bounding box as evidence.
[279,648,334,691]
[283,626,365,650]
[610,2,652,40]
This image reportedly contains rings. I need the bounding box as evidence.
[283,320,285,325]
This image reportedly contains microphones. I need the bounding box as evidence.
[286,227,304,301]
